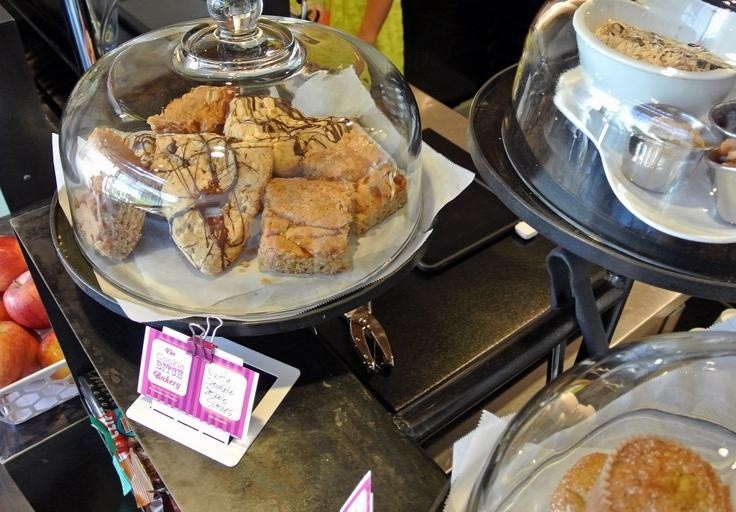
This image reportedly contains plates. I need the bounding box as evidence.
[489,407,735,512]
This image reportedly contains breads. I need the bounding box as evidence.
[595,15,730,72]
[74,85,406,275]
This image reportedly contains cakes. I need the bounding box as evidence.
[547,432,736,512]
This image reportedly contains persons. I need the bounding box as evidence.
[288,1,407,93]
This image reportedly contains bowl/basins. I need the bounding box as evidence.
[573,0,734,114]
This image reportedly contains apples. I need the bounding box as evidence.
[0,235,69,392]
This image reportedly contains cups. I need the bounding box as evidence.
[623,100,735,229]
